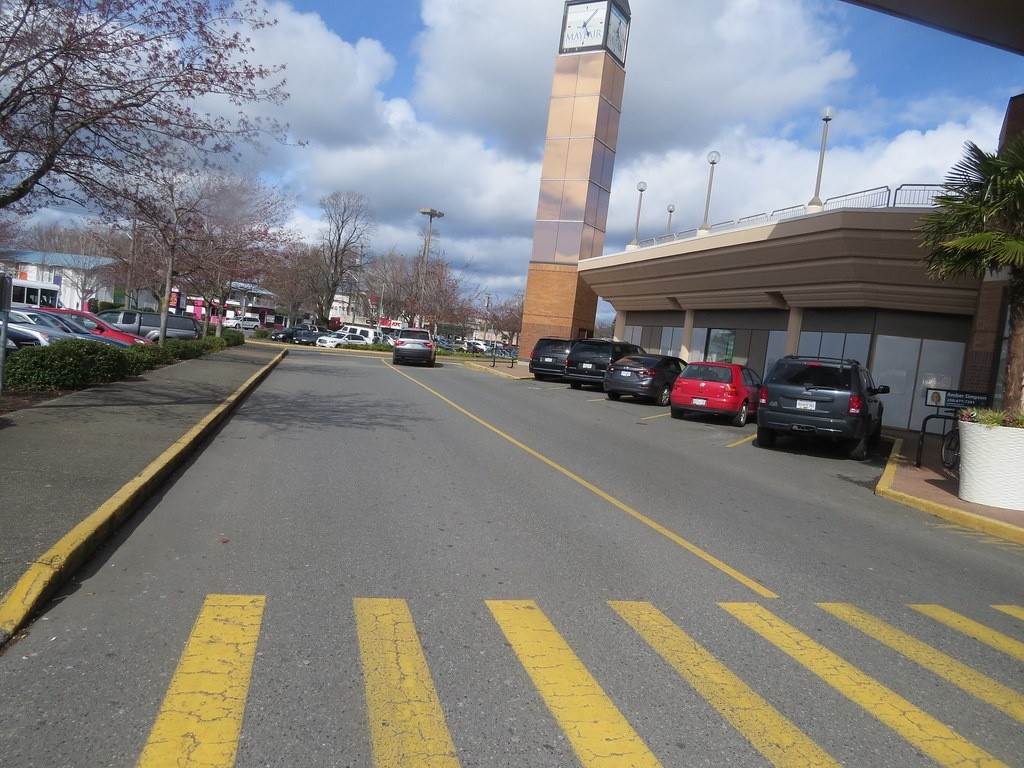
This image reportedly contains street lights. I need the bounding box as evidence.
[418,207,445,328]
[622,182,647,245]
[666,203,675,242]
[694,149,721,230]
[803,105,835,206]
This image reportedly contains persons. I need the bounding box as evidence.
[928,392,944,406]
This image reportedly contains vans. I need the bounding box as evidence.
[335,325,384,346]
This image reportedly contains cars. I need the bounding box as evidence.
[602,353,688,407]
[382,330,519,358]
[316,331,369,348]
[222,315,261,331]
[670,360,763,427]
[0,301,158,357]
[271,323,334,346]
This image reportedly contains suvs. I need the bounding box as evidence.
[392,327,440,368]
[562,337,648,393]
[755,353,891,462]
[528,334,582,384]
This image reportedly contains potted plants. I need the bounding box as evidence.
[957,407,1024,512]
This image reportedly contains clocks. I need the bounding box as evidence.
[562,1,608,49]
[606,3,630,64]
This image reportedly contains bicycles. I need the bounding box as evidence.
[941,410,960,469]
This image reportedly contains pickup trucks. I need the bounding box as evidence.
[71,308,199,344]
